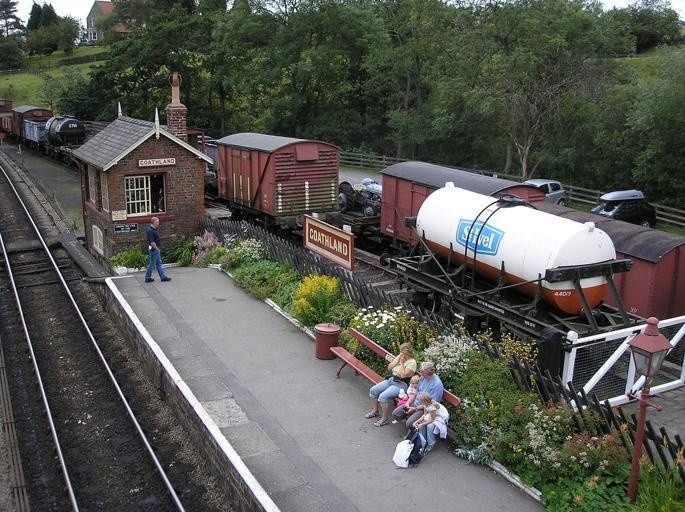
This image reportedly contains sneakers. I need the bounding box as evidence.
[391,419,405,424]
[145,277,154,282]
[399,388,405,399]
[426,442,436,452]
[374,417,390,426]
[365,411,379,418]
[161,277,171,281]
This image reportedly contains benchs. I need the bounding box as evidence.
[329,327,462,414]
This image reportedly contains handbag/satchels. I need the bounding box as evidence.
[393,440,414,468]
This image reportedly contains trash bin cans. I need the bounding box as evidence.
[313,322,341,360]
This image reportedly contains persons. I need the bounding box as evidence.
[364,342,450,457]
[145,217,172,282]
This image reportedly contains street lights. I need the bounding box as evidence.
[626,316,674,501]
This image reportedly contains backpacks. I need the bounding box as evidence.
[404,427,427,467]
